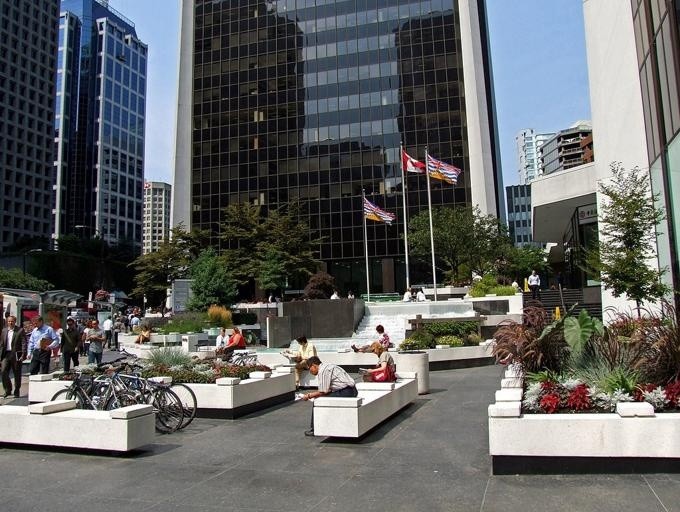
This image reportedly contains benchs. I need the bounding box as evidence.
[313,372,418,438]
[0,396,156,452]
[265,364,319,388]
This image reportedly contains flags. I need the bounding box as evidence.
[363,195,396,227]
[423,150,462,186]
[400,147,426,176]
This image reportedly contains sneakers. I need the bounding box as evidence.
[13,393,19,398]
[3,391,11,397]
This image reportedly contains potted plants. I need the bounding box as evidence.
[396,337,429,395]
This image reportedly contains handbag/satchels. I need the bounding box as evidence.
[16,351,27,361]
[40,337,53,350]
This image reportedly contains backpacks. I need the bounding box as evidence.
[373,364,397,382]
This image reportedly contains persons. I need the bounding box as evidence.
[362,341,397,383]
[416,287,426,301]
[0,305,160,375]
[291,334,318,392]
[268,292,273,302]
[351,324,390,354]
[348,290,355,298]
[221,326,246,361]
[300,356,358,436]
[527,270,542,301]
[402,288,414,302]
[330,290,341,299]
[216,327,230,358]
[0,315,28,398]
[556,271,565,289]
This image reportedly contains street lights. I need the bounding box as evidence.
[75,223,107,290]
[18,248,43,289]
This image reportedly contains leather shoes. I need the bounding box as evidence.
[305,431,313,436]
[351,345,359,352]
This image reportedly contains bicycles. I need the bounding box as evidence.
[50,356,198,434]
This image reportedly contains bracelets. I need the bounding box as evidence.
[307,393,311,399]
